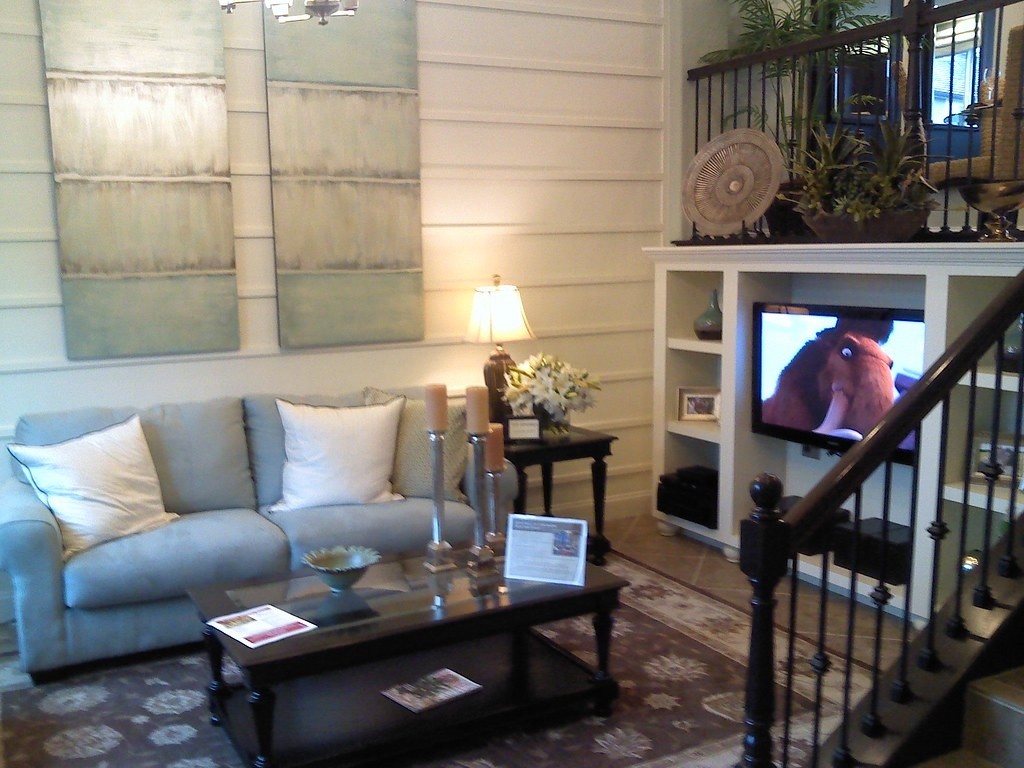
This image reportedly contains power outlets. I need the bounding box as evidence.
[801,444,820,461]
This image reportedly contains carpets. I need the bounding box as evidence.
[0,545,887,768]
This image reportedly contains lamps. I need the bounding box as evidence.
[218,0,359,26]
[464,274,537,444]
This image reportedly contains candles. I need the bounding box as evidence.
[426,384,448,430]
[485,422,505,473]
[467,385,490,433]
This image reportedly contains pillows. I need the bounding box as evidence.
[267,394,407,513]
[4,412,181,564]
[361,384,473,503]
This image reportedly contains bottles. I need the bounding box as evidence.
[694,289,722,340]
[994,313,1023,372]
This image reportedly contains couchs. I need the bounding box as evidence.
[0,384,519,688]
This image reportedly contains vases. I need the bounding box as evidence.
[541,410,572,442]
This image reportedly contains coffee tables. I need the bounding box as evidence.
[187,541,630,767]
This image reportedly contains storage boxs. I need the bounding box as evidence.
[774,495,850,557]
[834,517,911,586]
[657,465,720,530]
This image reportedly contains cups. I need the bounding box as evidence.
[958,180,1023,242]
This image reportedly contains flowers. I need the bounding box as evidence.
[497,350,603,435]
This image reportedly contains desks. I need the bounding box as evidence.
[467,425,621,566]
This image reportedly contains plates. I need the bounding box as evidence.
[683,129,784,236]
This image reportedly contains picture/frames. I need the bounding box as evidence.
[503,414,544,444]
[676,385,722,423]
[964,429,1024,489]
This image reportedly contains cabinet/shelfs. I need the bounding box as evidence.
[647,240,1024,629]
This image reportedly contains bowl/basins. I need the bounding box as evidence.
[301,546,381,592]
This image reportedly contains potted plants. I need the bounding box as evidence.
[771,114,969,243]
[697,0,936,237]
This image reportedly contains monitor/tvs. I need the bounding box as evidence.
[752,301,925,464]
